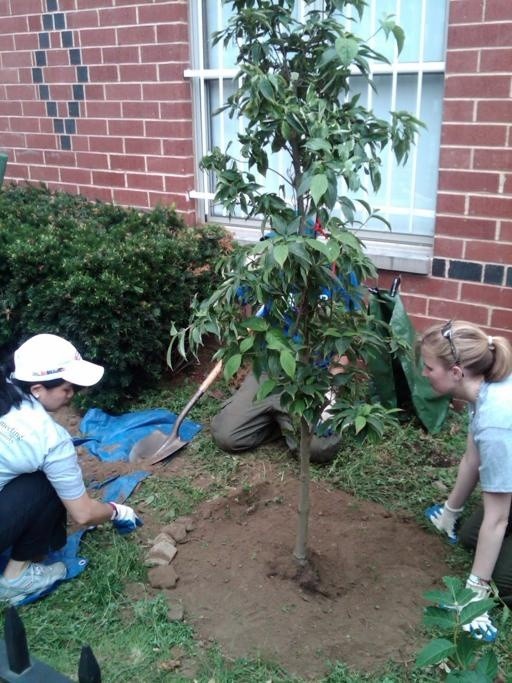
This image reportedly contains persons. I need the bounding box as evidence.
[413,318,512,642]
[206,211,368,467]
[0,330,145,600]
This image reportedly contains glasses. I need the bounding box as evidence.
[441,320,465,377]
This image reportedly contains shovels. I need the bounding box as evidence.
[130,327,252,466]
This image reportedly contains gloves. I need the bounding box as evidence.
[109,501,144,535]
[439,579,498,642]
[424,501,465,543]
[314,386,337,438]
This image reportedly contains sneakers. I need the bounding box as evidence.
[0,560,66,604]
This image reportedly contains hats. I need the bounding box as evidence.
[259,211,315,241]
[11,334,104,388]
